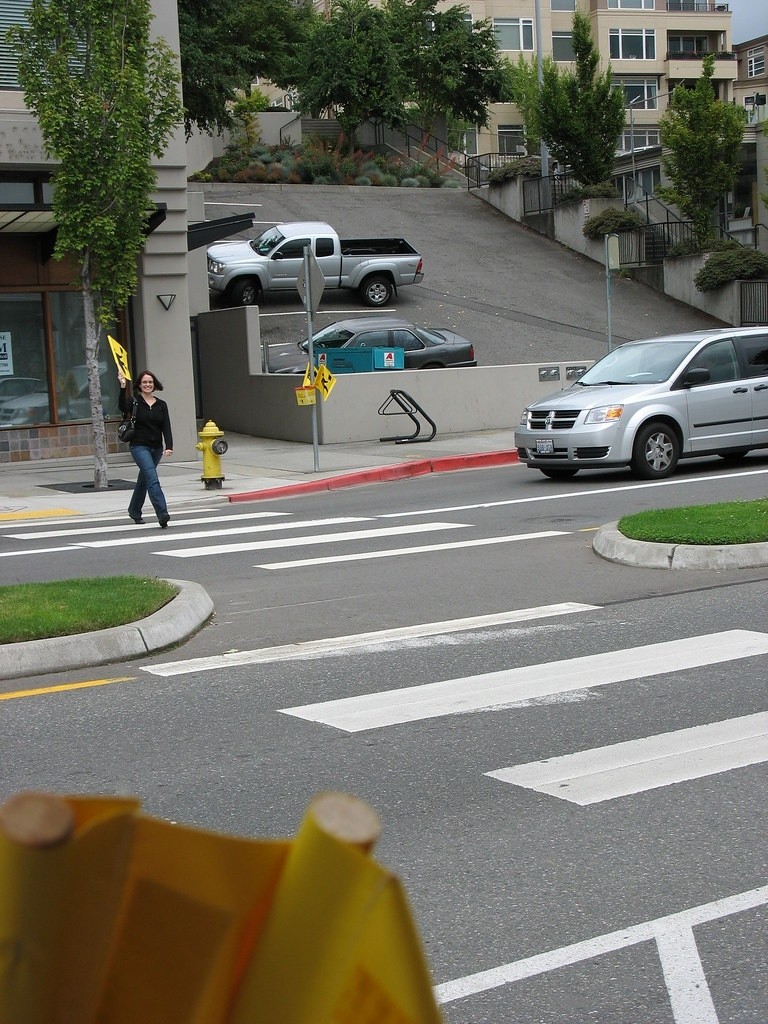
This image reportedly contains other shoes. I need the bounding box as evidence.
[129,515,145,524]
[160,513,170,527]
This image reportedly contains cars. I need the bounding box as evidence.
[0,360,112,427]
[261,315,478,374]
[0,376,41,404]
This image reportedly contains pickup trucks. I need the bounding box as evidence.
[207,220,424,308]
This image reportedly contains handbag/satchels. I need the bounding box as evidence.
[117,397,138,442]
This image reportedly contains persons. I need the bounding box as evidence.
[118,371,173,527]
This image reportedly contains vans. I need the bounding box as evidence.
[514,325,768,481]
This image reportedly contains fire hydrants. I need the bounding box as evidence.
[196,418,228,490]
